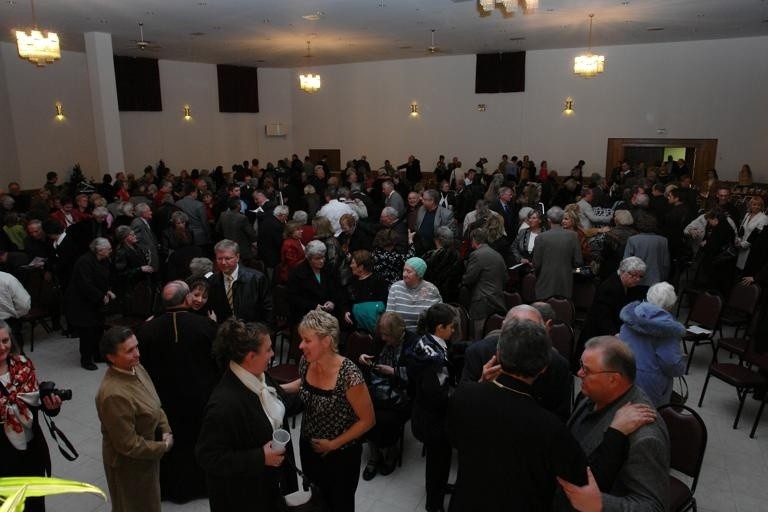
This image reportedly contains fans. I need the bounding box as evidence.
[114,18,167,54]
[406,26,455,58]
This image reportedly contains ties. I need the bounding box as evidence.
[226,276,235,316]
[504,204,511,214]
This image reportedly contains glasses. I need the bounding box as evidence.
[578,359,624,376]
[628,271,645,280]
[129,233,135,237]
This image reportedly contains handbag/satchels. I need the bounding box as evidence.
[361,343,409,413]
[589,254,601,276]
[460,224,471,257]
[450,169,457,190]
[274,453,330,512]
[707,221,738,270]
[671,375,690,414]
[614,200,626,210]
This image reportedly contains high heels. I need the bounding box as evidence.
[379,451,402,476]
[362,451,385,481]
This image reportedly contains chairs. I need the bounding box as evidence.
[0,243,768,470]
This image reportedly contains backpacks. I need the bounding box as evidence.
[666,228,693,267]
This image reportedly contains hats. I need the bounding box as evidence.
[405,256,428,278]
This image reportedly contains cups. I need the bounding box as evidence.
[271,427,291,451]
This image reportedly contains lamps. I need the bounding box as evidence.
[52,96,67,122]
[293,39,325,97]
[180,103,193,125]
[561,96,575,116]
[7,0,66,71]
[408,100,420,120]
[570,11,607,81]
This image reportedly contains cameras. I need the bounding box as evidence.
[38,381,73,401]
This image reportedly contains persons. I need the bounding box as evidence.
[0,153,767,512]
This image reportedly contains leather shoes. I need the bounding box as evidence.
[80,357,99,371]
[63,328,82,339]
